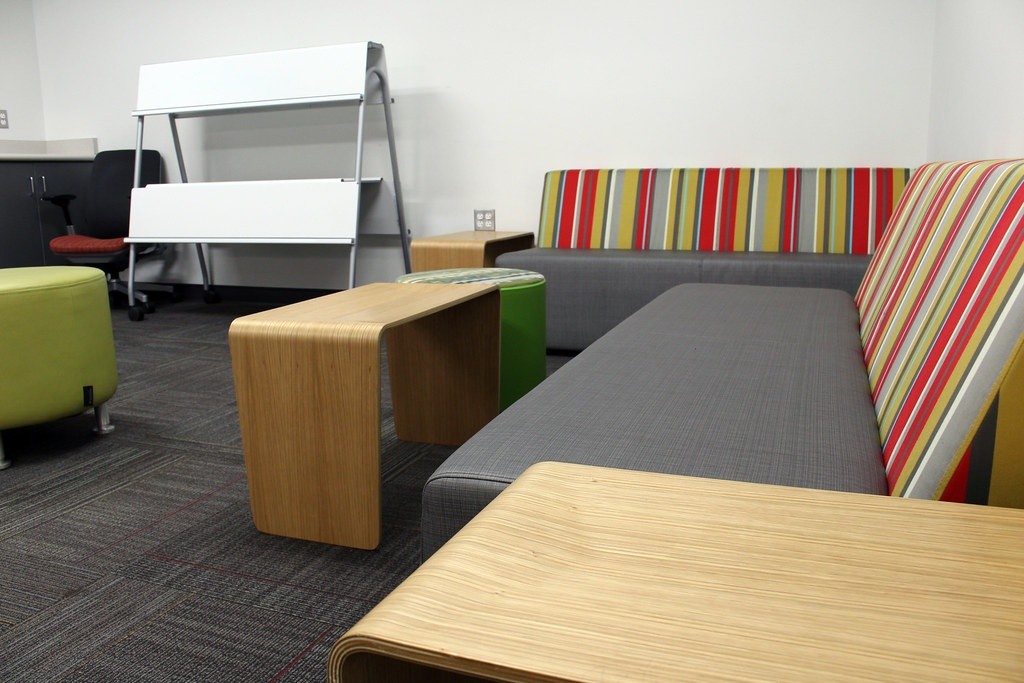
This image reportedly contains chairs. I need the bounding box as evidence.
[41,149,188,314]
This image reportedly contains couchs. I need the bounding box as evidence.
[417,152,1024,561]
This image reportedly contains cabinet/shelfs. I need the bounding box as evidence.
[0,156,94,269]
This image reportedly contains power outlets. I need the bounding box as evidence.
[474,209,496,232]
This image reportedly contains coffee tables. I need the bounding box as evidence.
[410,229,535,271]
[228,282,502,550]
[323,462,1024,683]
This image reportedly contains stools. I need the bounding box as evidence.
[1,266,118,469]
[394,268,547,412]
